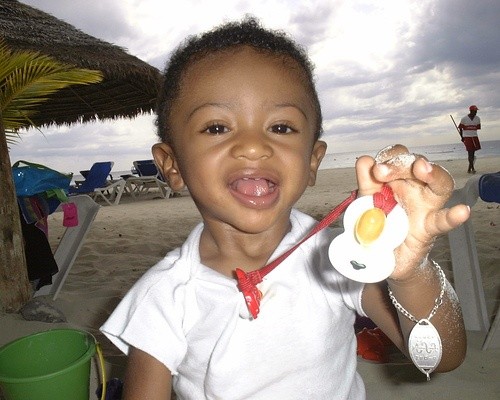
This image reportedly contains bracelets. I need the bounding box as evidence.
[386,258,446,381]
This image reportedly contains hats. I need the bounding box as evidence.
[470,105,479,110]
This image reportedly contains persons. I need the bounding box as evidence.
[98,21,471,399]
[458,104,482,174]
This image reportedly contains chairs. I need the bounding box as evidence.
[70,160,180,206]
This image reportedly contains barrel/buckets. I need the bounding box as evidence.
[63,198,78,227]
[0,329,106,400]
